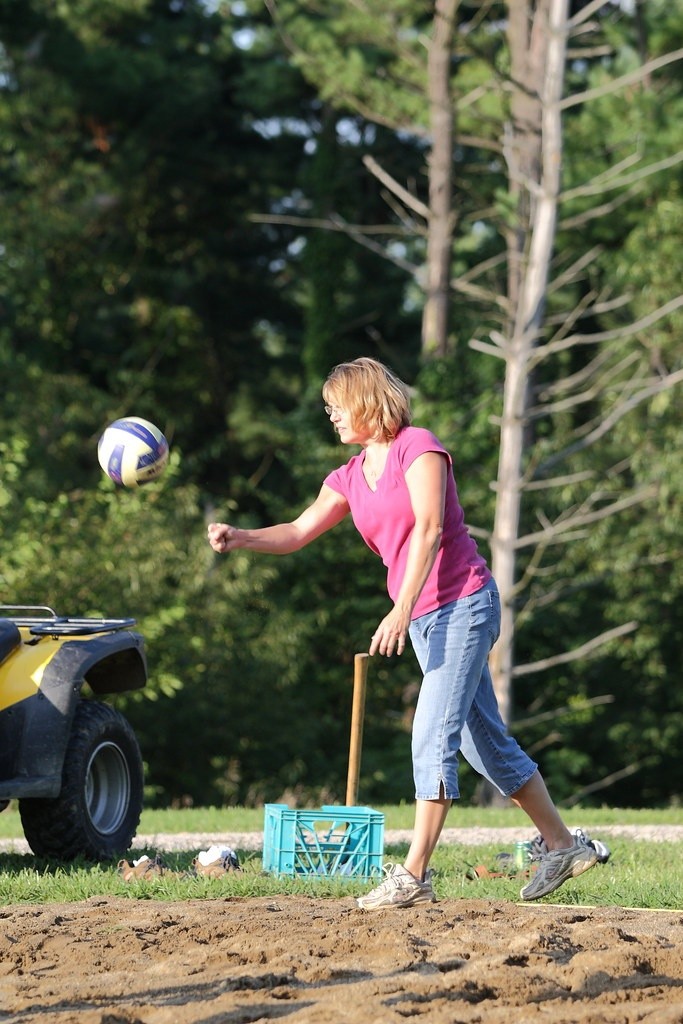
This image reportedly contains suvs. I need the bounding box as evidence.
[1,603,149,861]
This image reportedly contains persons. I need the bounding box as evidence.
[208,358,600,907]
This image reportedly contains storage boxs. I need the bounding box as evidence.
[262,804,384,884]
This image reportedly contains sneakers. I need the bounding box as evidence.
[354,861,437,911]
[521,829,601,901]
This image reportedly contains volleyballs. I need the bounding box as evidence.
[95,415,172,489]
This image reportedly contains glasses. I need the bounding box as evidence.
[325,405,346,415]
